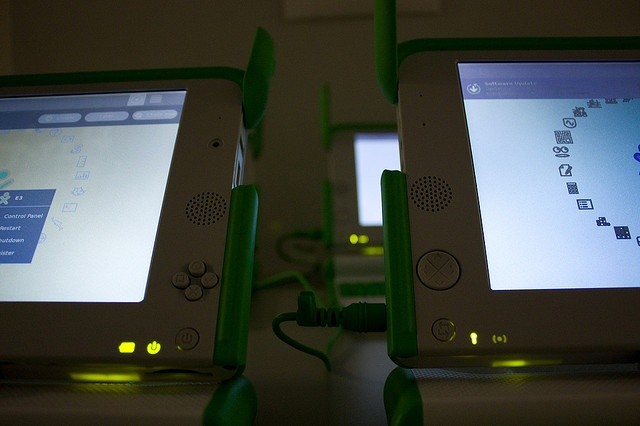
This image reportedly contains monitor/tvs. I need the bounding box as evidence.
[371,3,640,426]
[319,85,403,279]
[1,25,278,425]
